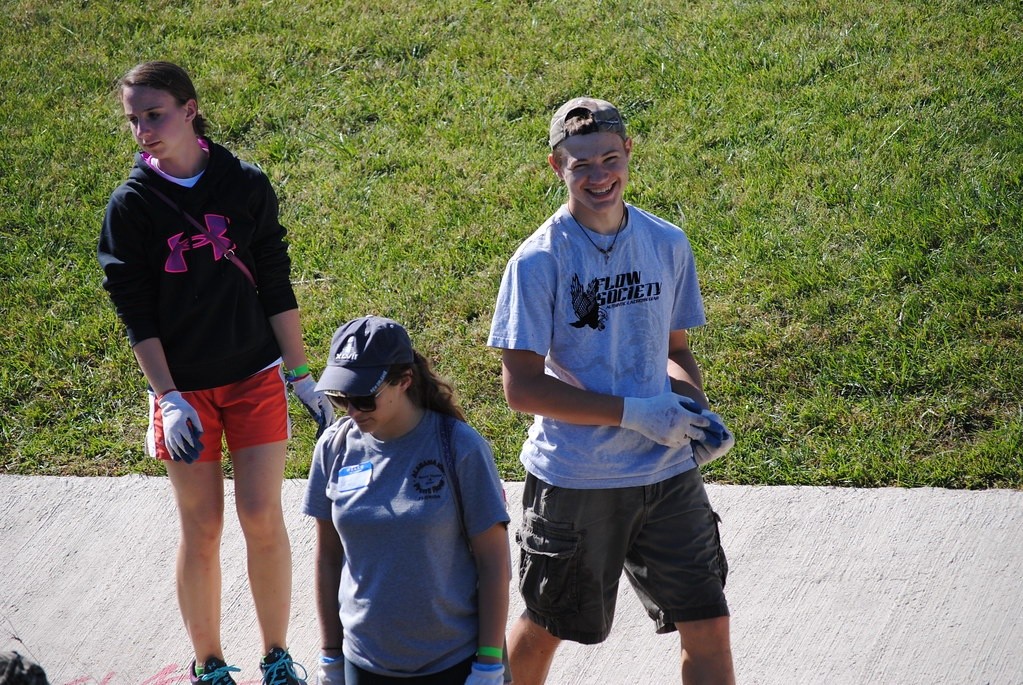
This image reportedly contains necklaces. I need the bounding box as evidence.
[568,201,625,266]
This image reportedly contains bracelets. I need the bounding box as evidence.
[321,647,341,650]
[283,363,309,381]
[477,647,503,660]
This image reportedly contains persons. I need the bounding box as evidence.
[97,61,334,685]
[304,314,513,685]
[485,97,735,685]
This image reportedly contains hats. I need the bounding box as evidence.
[313,314,414,397]
[548,97,624,149]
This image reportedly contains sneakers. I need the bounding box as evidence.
[260,647,310,685]
[189,655,241,685]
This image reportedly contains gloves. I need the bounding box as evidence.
[155,389,205,464]
[618,394,710,446]
[287,373,335,439]
[466,662,501,685]
[693,407,734,468]
[318,656,342,685]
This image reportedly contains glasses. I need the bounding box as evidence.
[325,374,395,412]
